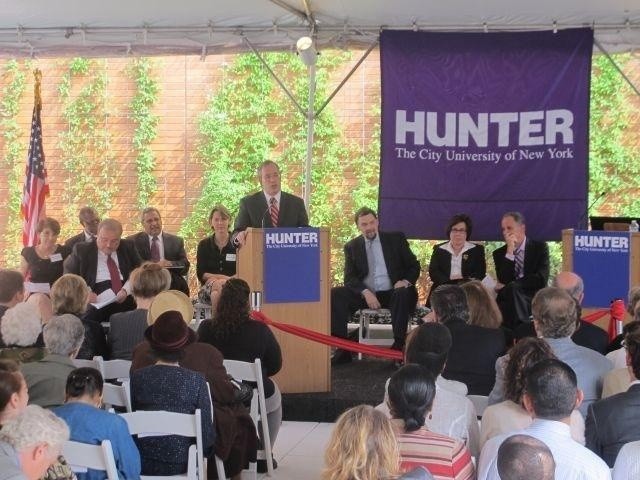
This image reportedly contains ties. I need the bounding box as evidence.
[150,235,160,263]
[107,254,122,295]
[513,248,525,279]
[269,197,279,229]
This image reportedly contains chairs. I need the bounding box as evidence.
[224,357,274,477]
[110,407,208,480]
[64,440,120,480]
[75,355,94,372]
[99,382,130,415]
[92,356,130,384]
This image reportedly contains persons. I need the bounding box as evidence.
[326,271,639,480]
[230,161,309,248]
[330,207,421,367]
[1,262,283,480]
[426,214,487,314]
[491,211,550,331]
[197,205,241,318]
[20,206,189,319]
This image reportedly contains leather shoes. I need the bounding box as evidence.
[331,349,350,365]
[390,356,403,371]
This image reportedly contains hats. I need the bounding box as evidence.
[406,322,452,379]
[145,310,198,350]
[147,290,194,326]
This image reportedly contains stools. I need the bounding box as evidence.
[358,310,411,362]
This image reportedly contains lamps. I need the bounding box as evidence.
[297,37,316,67]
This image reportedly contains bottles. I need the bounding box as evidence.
[629,220,639,231]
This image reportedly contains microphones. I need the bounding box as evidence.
[260,197,276,227]
[578,192,606,230]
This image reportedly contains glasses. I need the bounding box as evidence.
[620,340,629,350]
[82,219,101,227]
[530,315,544,324]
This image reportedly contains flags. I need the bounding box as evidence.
[21,105,50,282]
